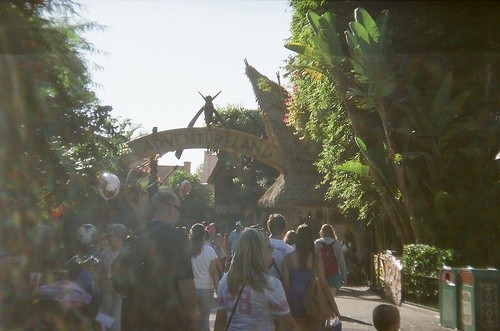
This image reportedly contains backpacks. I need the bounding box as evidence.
[318,240,338,277]
[112,223,169,296]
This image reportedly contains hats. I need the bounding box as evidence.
[235,221,241,225]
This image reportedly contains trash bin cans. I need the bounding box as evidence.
[438,266,462,330]
[458,267,500,331]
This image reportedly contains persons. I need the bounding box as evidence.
[63,190,352,331]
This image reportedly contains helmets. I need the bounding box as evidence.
[77,224,98,245]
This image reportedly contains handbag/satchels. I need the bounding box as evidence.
[302,252,340,322]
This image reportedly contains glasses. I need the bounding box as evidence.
[166,201,185,216]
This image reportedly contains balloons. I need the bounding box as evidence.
[180,181,190,194]
[97,172,121,202]
